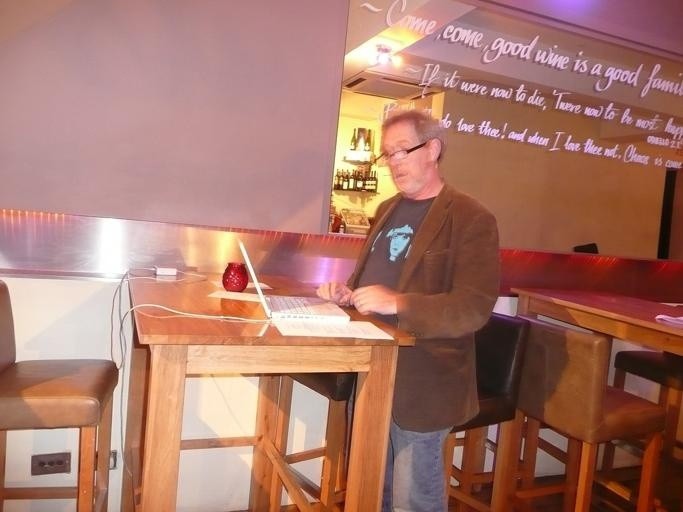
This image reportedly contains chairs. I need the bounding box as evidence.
[266,359,357,512]
[601,343,681,511]
[504,313,666,510]
[441,306,532,512]
[2,282,118,512]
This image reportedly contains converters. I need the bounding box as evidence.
[153,265,177,276]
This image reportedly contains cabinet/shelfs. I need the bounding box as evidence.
[332,125,380,194]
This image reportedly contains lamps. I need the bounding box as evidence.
[367,43,402,67]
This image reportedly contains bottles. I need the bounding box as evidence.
[349,128,371,151]
[333,168,377,193]
[328,216,333,232]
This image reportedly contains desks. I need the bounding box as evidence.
[504,283,683,511]
[121,266,418,511]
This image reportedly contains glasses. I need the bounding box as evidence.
[374,142,426,167]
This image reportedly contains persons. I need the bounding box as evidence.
[314,112,502,512]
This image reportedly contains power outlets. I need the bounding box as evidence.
[110,450,117,470]
[26,451,71,476]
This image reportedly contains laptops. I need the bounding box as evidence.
[237,239,350,325]
